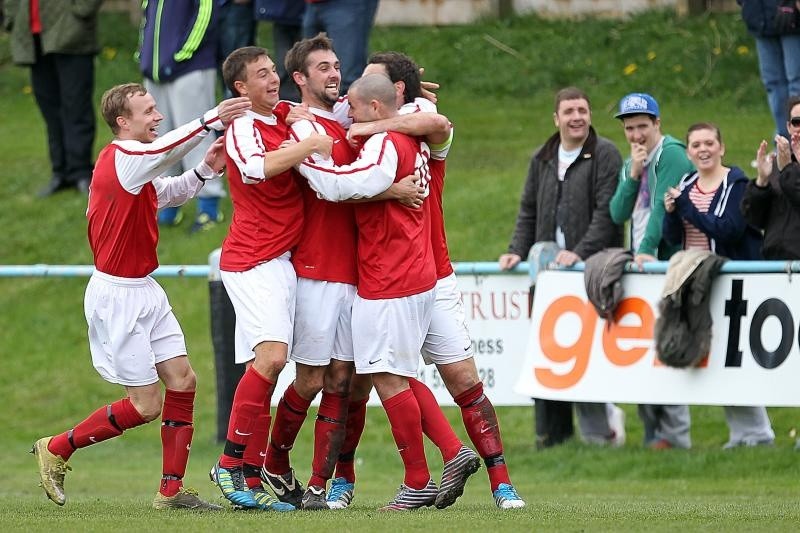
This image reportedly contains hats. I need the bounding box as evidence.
[612,93,659,120]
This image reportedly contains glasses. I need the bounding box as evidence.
[788,116,800,128]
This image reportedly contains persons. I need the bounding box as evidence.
[734,0,800,168]
[277,73,485,512]
[664,118,776,450]
[131,0,228,239]
[259,31,442,511]
[28,78,254,512]
[328,47,527,510]
[737,94,800,261]
[498,88,625,449]
[208,46,335,513]
[607,92,698,453]
[215,0,379,121]
[0,0,105,200]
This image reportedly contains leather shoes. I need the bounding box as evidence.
[36,178,70,198]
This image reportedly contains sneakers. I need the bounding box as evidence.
[259,463,305,507]
[209,457,258,509]
[155,211,182,228]
[326,478,355,509]
[493,483,526,510]
[377,478,440,510]
[184,211,225,233]
[250,487,297,513]
[434,446,480,509]
[30,436,66,506]
[300,485,330,511]
[152,490,225,511]
